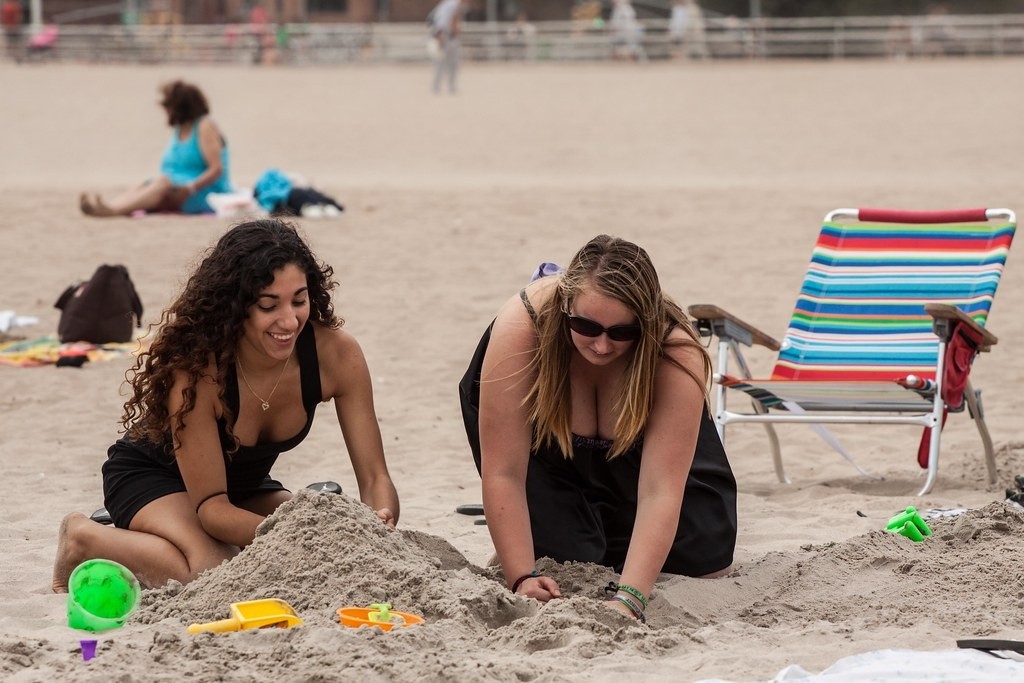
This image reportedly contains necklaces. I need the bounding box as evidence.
[237,356,289,411]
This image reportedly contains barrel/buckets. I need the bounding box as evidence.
[67,558,141,630]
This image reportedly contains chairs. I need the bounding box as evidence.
[688,206,1017,498]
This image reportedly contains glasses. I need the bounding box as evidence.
[564,298,642,343]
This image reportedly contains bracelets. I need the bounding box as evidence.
[196,492,228,514]
[609,586,646,624]
[512,567,541,594]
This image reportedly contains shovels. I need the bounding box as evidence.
[187,596,306,638]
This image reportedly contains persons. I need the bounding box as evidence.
[608,0,648,60]
[53,219,400,594]
[666,0,707,58]
[426,0,472,96]
[81,81,231,217]
[459,235,737,620]
